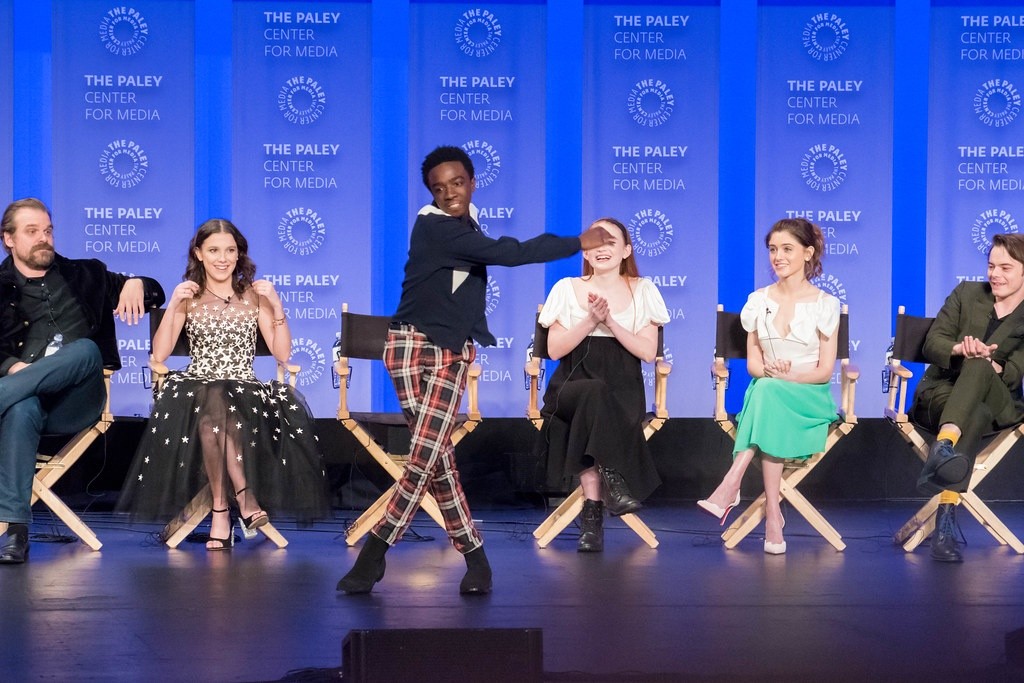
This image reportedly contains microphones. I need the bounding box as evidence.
[766,307,772,314]
[224,296,231,303]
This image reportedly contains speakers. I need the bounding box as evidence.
[341,627,544,683]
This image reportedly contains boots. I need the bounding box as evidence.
[336,532,390,594]
[460,544,492,595]
[929,503,961,561]
[598,465,643,516]
[577,499,604,552]
[916,438,970,497]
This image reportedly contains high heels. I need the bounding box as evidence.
[764,512,786,554]
[234,486,269,539]
[205,506,234,551]
[697,489,740,526]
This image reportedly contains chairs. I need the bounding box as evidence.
[337,303,480,543]
[0,368,114,550]
[884,304,1024,557]
[711,304,860,548]
[149,305,300,548]
[524,306,671,547]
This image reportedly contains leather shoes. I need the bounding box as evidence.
[0,524,31,563]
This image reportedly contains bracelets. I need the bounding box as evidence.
[272,314,286,326]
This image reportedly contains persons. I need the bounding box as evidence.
[336,146,611,597]
[541,221,671,553]
[153,218,291,550]
[696,216,840,556]
[914,234,1024,560]
[0,198,167,563]
